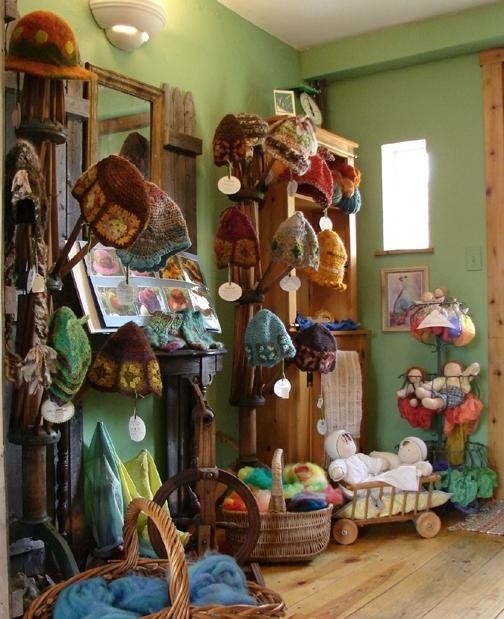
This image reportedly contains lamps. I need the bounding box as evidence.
[89,0,167,55]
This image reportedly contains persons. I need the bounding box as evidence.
[414,359,480,411]
[323,430,389,500]
[406,285,477,347]
[369,435,433,495]
[392,275,420,324]
[395,366,433,408]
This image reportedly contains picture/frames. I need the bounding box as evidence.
[380,266,430,332]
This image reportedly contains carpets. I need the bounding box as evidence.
[445,502,504,537]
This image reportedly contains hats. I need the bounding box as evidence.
[244,310,297,369]
[272,212,348,291]
[43,306,93,408]
[4,10,99,80]
[71,156,193,272]
[295,322,336,373]
[212,114,335,209]
[6,140,46,225]
[216,207,259,268]
[88,322,164,398]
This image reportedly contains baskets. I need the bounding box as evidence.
[218,450,334,564]
[22,499,286,619]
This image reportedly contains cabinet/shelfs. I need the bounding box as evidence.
[235,123,373,469]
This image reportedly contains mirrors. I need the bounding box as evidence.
[83,63,164,192]
